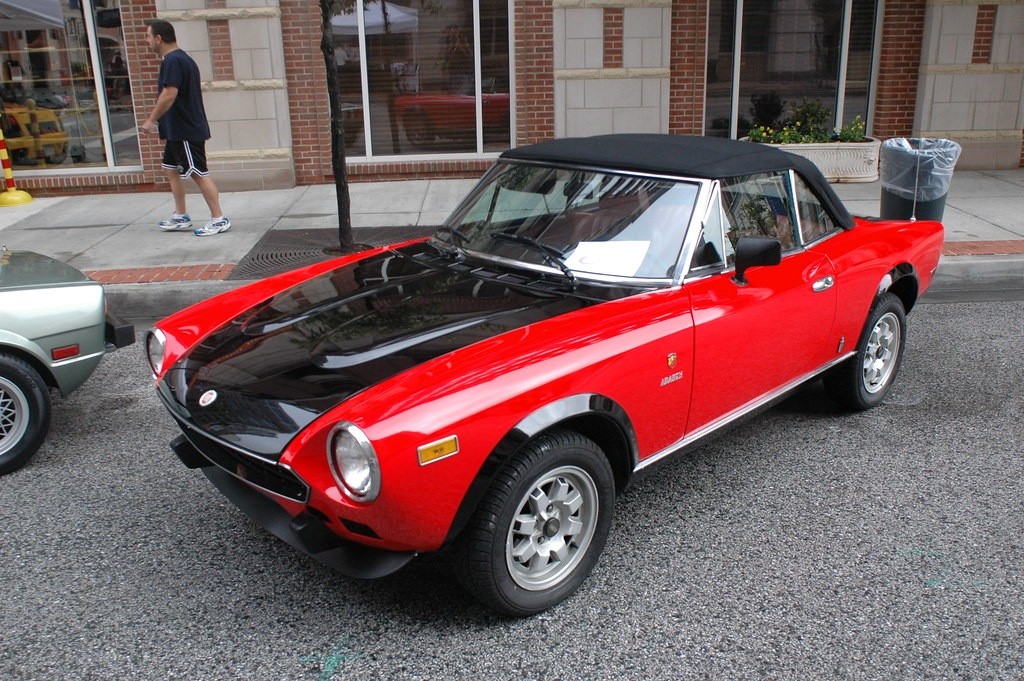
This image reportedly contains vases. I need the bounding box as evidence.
[737,137,882,183]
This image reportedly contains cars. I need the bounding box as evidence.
[0,241,139,475]
[141,131,947,621]
[337,99,365,146]
[396,64,510,149]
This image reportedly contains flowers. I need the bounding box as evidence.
[747,97,869,143]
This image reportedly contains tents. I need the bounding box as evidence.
[0,0,64,32]
[332,0,419,97]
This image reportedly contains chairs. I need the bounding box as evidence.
[567,210,628,246]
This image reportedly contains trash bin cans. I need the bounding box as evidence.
[879,136,962,222]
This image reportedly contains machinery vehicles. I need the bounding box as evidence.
[0,79,71,167]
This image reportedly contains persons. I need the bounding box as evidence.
[334,42,359,72]
[141,19,231,237]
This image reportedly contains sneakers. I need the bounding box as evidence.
[194,215,231,237]
[159,211,192,231]
